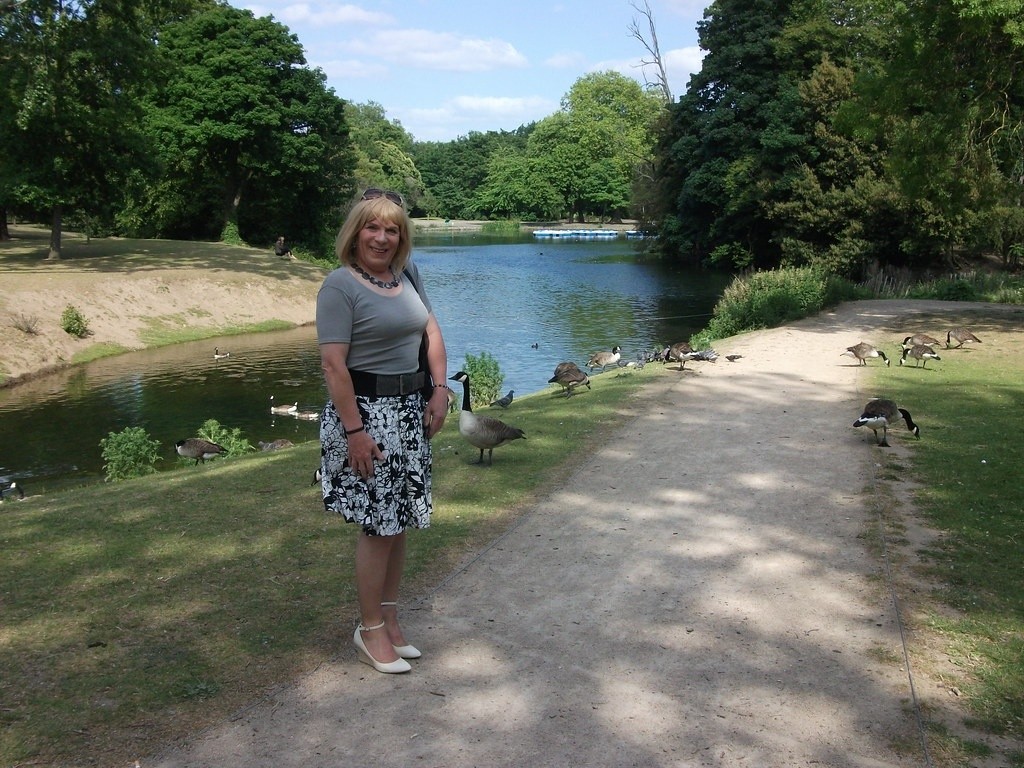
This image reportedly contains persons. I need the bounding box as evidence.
[275,236,297,259]
[316,189,449,673]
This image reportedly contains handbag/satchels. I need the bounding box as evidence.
[417,337,434,403]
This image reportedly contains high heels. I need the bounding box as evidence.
[353,619,412,674]
[380,601,421,659]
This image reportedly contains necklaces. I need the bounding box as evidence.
[352,263,400,288]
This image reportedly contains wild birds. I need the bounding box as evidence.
[617,342,745,371]
[174,438,222,466]
[554,362,578,392]
[900,345,941,368]
[203,441,229,462]
[548,369,591,399]
[270,401,298,416]
[0,481,24,499]
[853,399,920,447]
[531,342,539,349]
[214,347,230,360]
[490,390,515,409]
[902,334,940,350]
[258,439,294,451]
[839,341,890,367]
[946,327,983,348]
[294,410,320,421]
[585,346,622,373]
[448,372,527,465]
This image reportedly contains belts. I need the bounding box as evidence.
[348,368,426,397]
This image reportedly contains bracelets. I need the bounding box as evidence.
[346,424,364,434]
[432,384,449,390]
